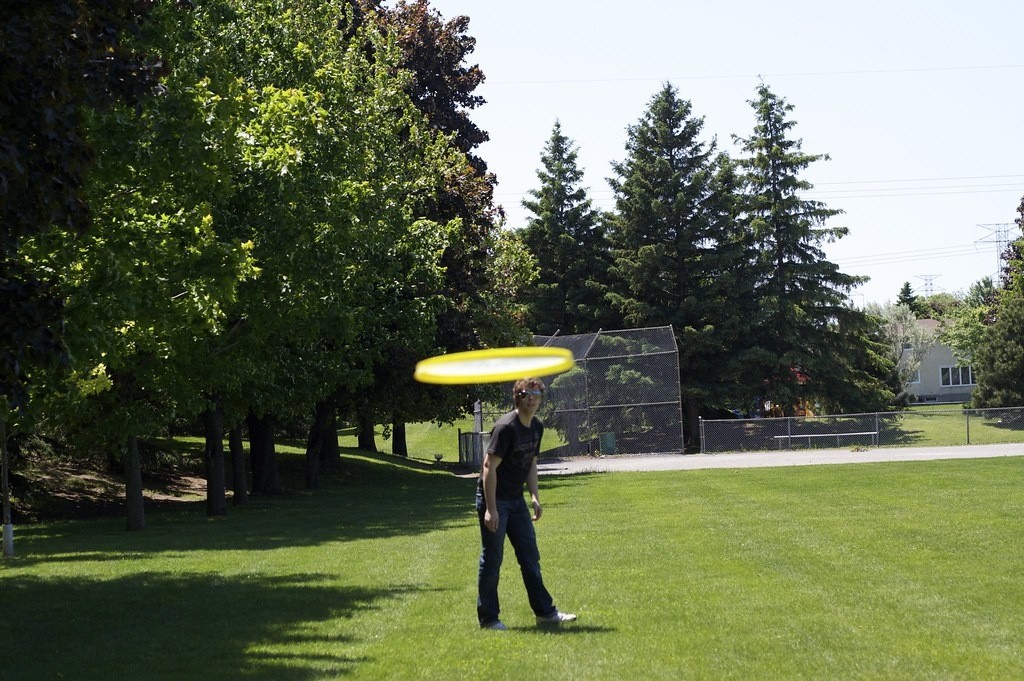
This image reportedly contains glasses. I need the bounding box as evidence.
[518,389,542,399]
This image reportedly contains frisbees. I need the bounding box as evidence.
[414,346,575,385]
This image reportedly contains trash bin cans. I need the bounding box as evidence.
[598,432,617,455]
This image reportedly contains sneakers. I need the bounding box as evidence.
[481,621,506,630]
[536,610,577,626]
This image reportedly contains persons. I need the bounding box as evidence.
[475,377,577,630]
